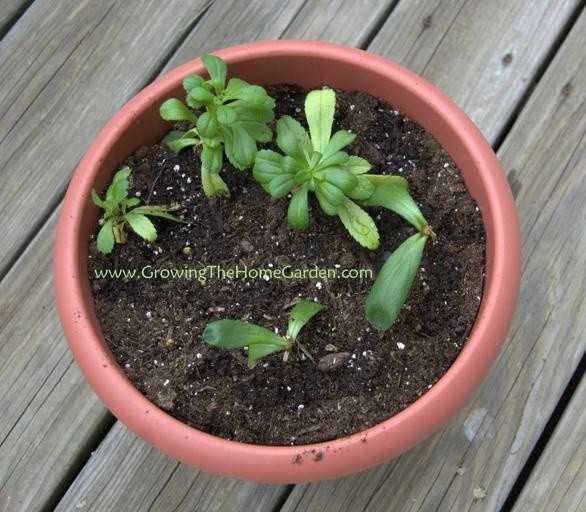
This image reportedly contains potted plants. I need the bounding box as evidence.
[53,38,521,484]
